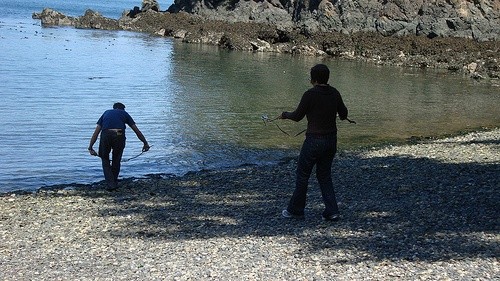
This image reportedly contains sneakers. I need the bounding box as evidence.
[321,214,340,221]
[282,210,305,219]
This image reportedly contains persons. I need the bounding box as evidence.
[271,64,349,221]
[88,102,149,190]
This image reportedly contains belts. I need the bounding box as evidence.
[104,129,124,133]
